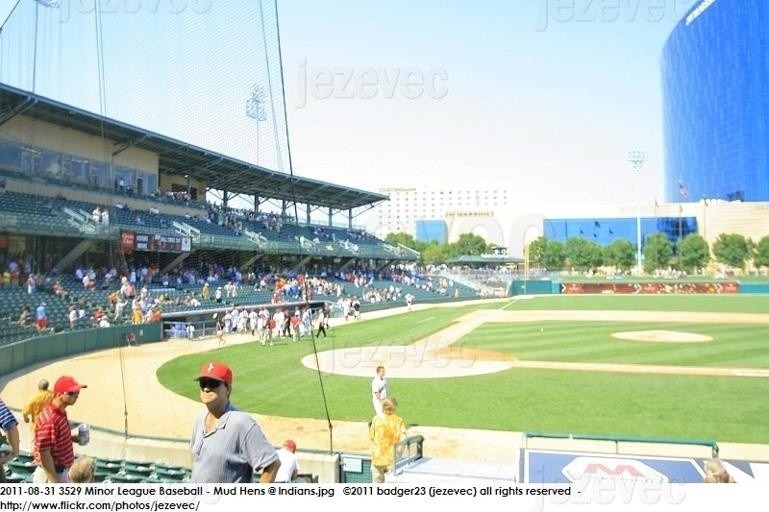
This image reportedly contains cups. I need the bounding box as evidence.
[78,423,90,446]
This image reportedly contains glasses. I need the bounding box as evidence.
[198,381,224,389]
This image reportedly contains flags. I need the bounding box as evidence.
[678,177,689,197]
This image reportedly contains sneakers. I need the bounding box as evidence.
[226,329,313,347]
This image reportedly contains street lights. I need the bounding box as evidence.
[625,145,649,275]
[246,83,267,166]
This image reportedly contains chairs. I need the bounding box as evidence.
[0,178,490,356]
[0,450,321,485]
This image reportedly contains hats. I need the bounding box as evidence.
[54,376,88,393]
[195,361,233,384]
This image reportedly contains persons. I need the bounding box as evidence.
[0,176,547,483]
